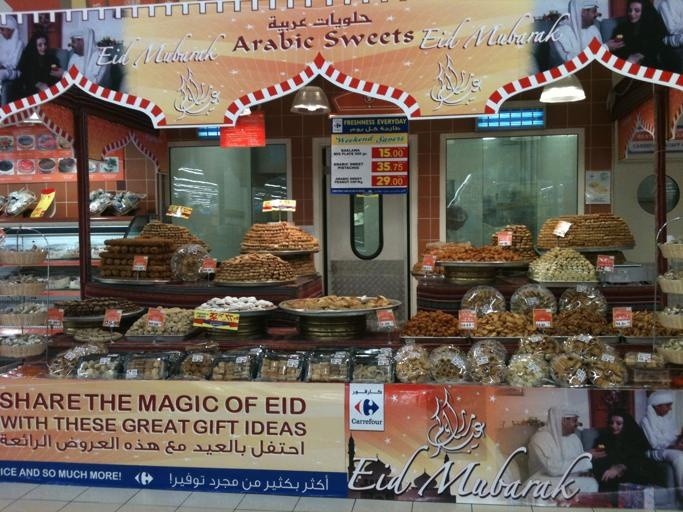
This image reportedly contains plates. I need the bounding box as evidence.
[0,161,14,174]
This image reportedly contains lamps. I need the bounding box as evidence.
[539,74,586,103]
[290,86,331,115]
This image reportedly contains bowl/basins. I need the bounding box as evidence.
[37,159,56,173]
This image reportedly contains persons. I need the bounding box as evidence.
[639,391,683,487]
[591,410,658,489]
[616,0,666,65]
[546,1,626,73]
[527,406,605,492]
[651,0,683,73]
[0,15,123,108]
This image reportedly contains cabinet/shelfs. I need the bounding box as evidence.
[0,258,102,302]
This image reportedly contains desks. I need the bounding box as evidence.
[416,279,662,320]
[85,273,322,328]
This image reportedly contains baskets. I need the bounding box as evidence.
[0,248,46,360]
[657,241,682,365]
